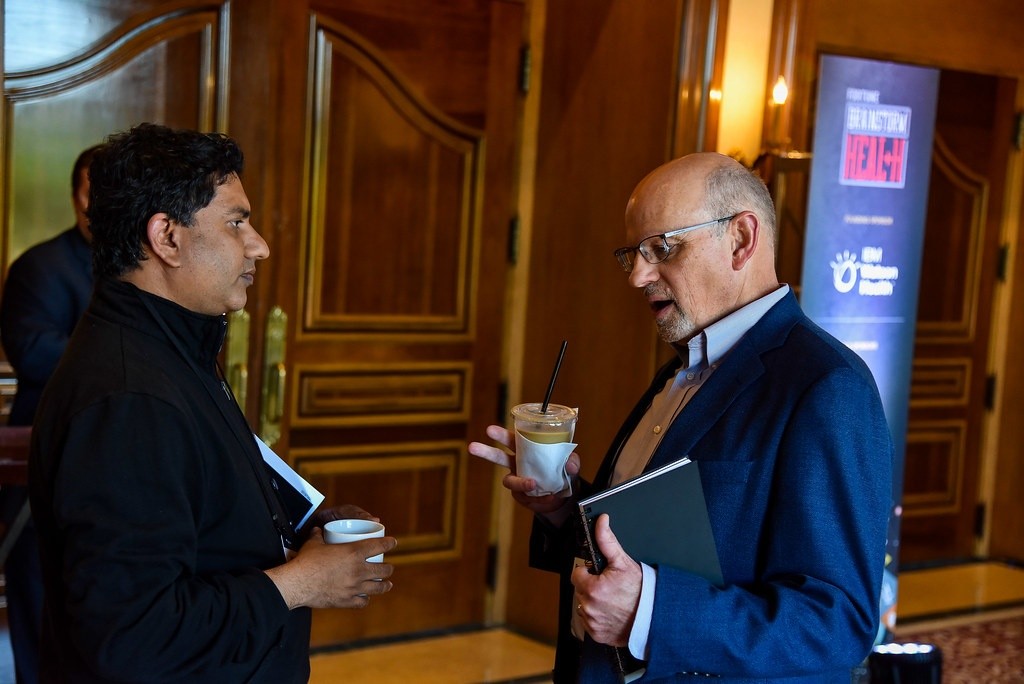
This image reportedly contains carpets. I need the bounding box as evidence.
[509,612,1024,684]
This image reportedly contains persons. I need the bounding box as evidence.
[0,122,401,684]
[466,152,897,684]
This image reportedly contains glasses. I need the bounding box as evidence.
[614,215,735,273]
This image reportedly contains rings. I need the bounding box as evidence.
[576,603,583,617]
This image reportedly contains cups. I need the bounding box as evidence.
[509,403,578,497]
[323,518,386,596]
